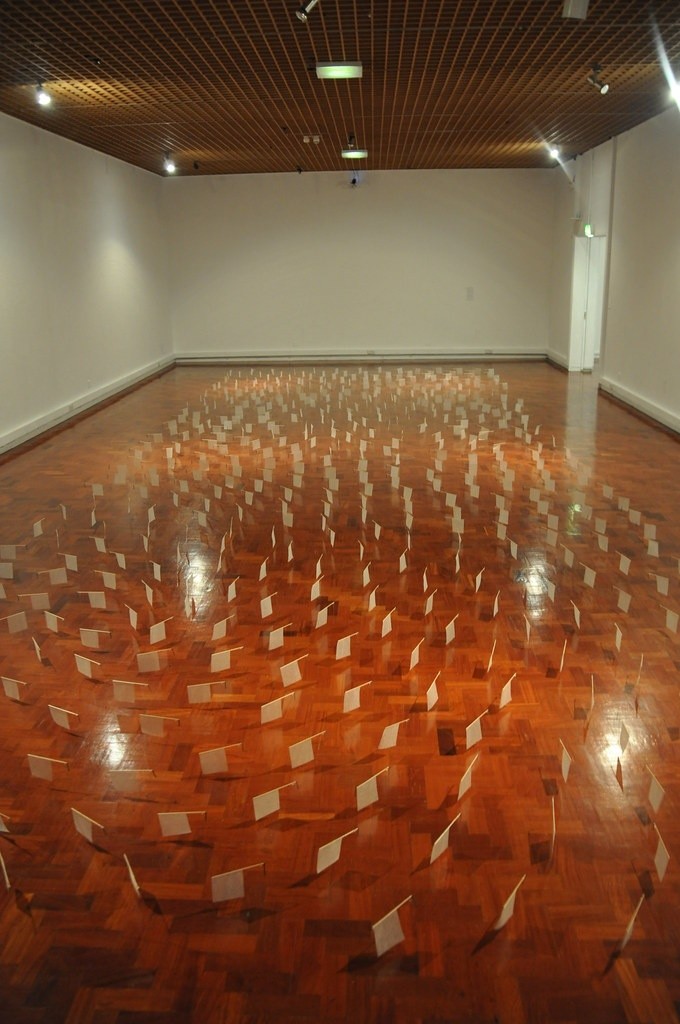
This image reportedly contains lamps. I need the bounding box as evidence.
[587,64,609,95]
[294,0,318,23]
[347,135,356,148]
